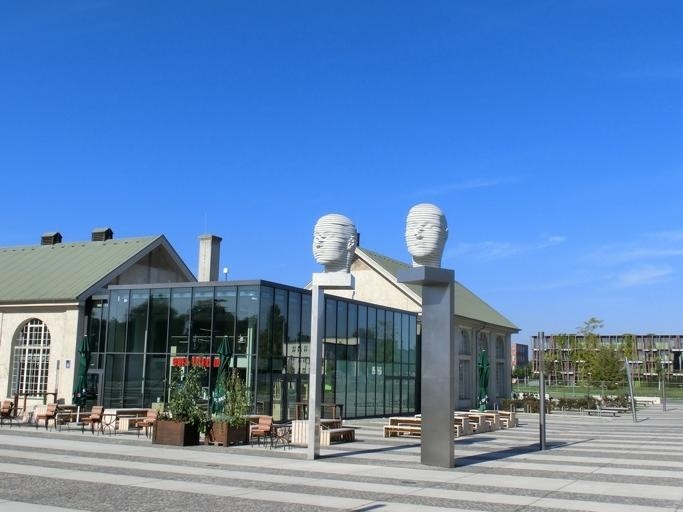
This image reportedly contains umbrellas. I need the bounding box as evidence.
[210,331,231,414]
[474,346,489,413]
[72,334,91,424]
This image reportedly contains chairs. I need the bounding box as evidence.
[0,399,293,452]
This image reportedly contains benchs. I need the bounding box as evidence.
[383,410,518,440]
[585,407,629,417]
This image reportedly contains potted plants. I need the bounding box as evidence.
[152,365,251,447]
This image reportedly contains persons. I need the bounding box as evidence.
[405,203,447,269]
[311,214,357,275]
